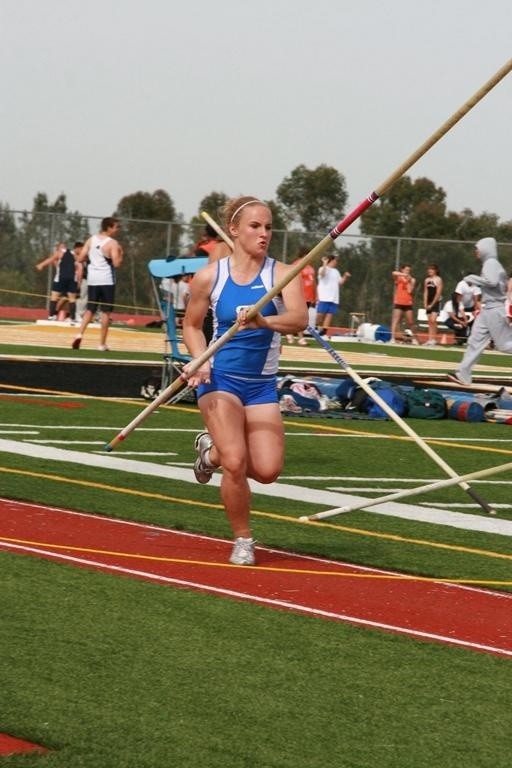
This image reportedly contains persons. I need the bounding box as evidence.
[439,271,511,345]
[284,245,352,344]
[446,238,512,385]
[180,197,308,568]
[173,274,195,318]
[36,242,104,325]
[195,224,235,265]
[389,264,445,345]
[70,216,126,353]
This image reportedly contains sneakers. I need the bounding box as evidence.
[448,372,463,384]
[73,335,82,350]
[193,432,214,484]
[228,536,256,566]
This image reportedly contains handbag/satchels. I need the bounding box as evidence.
[351,380,447,421]
